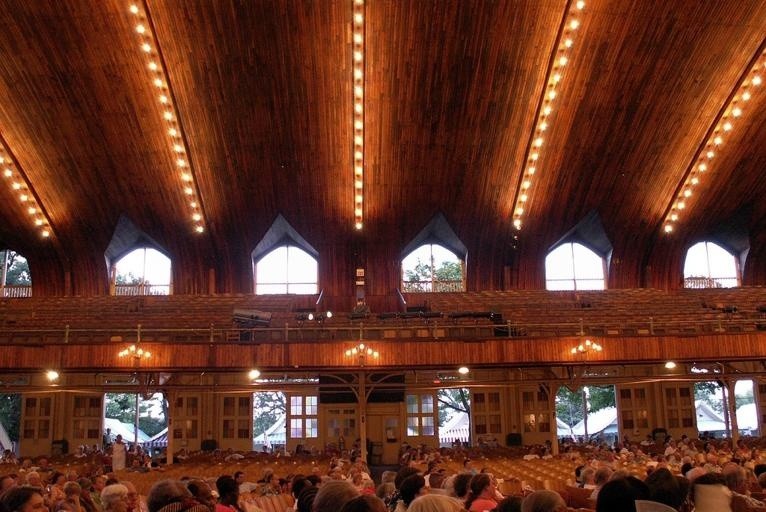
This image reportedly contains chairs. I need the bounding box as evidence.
[1,434,765,511]
[1,286,766,343]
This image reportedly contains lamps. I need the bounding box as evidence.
[571,339,603,354]
[345,343,379,358]
[118,344,151,359]
